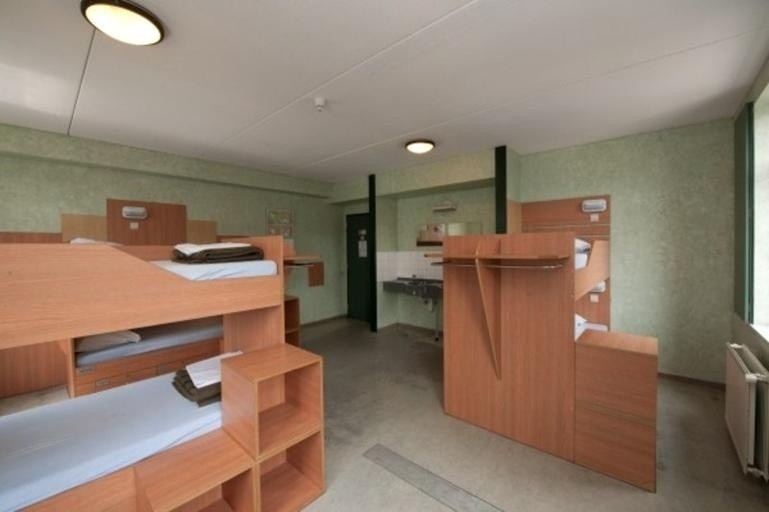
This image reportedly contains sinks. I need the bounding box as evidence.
[405,280,436,286]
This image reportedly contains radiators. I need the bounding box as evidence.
[721,340,769,485]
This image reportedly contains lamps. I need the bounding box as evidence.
[78,1,166,48]
[405,139,435,155]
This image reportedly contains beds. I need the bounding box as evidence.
[64,295,301,399]
[0,344,283,511]
[442,193,611,465]
[1,234,283,350]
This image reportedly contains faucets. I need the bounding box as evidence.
[411,274,415,281]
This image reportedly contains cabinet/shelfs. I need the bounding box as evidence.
[134,344,326,511]
